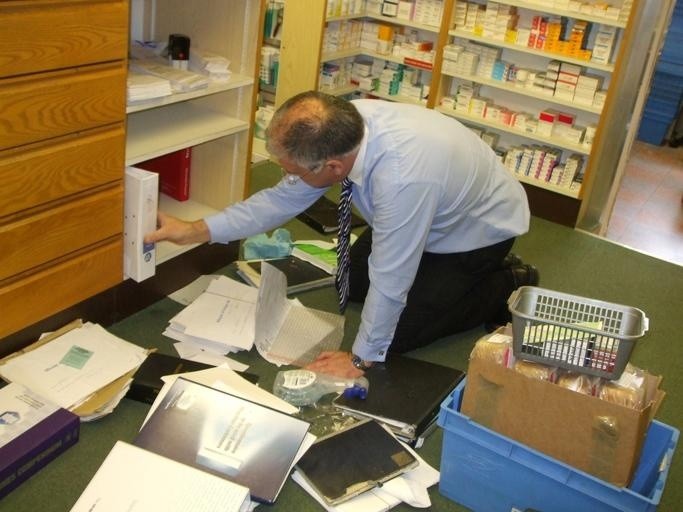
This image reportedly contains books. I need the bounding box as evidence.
[127,59,209,102]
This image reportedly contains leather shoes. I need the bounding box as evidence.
[507,253,540,291]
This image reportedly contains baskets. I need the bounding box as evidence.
[507,285,650,381]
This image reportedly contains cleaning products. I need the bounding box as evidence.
[273,368,369,406]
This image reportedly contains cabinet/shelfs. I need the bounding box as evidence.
[124,0,269,289]
[318,0,657,230]
[2,1,127,340]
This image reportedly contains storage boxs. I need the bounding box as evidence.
[437,377,679,512]
[459,323,666,491]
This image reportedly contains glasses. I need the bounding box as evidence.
[289,167,317,185]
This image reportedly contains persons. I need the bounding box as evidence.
[143,91,539,380]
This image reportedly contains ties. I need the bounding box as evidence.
[335,176,354,315]
